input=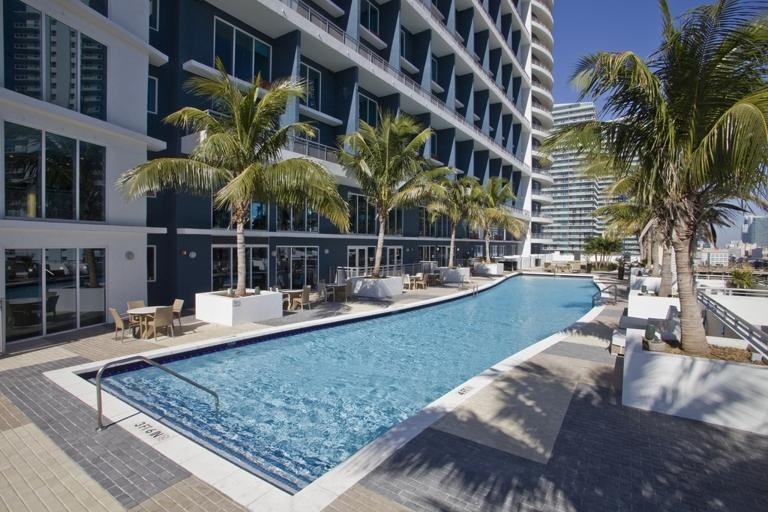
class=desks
[6,297,48,326]
[276,288,304,309]
[326,281,348,301]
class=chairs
[402,272,429,290]
[291,285,311,313]
[40,291,60,324]
[108,298,185,344]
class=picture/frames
[147,243,159,284]
